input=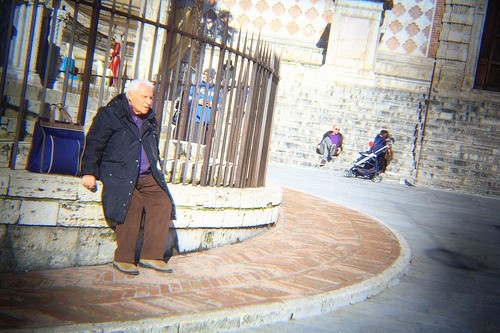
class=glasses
[334,128,340,131]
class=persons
[185,68,222,145]
[316,123,344,167]
[80,78,177,276]
[110,33,122,86]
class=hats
[204,68,216,78]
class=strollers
[343,129,395,183]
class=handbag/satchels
[27,103,85,177]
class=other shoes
[319,159,326,166]
[330,144,336,156]
[139,258,173,274]
[113,260,139,275]
